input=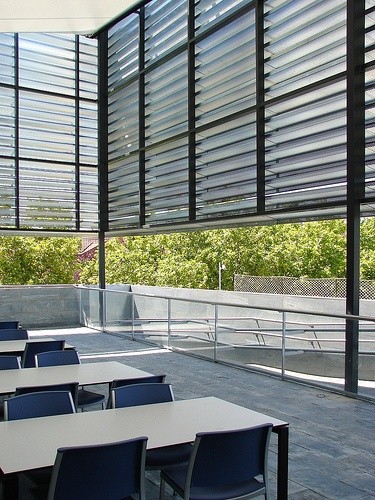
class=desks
[0,360,156,394]
[0,338,77,353]
[0,396,290,500]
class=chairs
[159,422,273,500]
[0,320,200,421]
[47,436,149,500]
